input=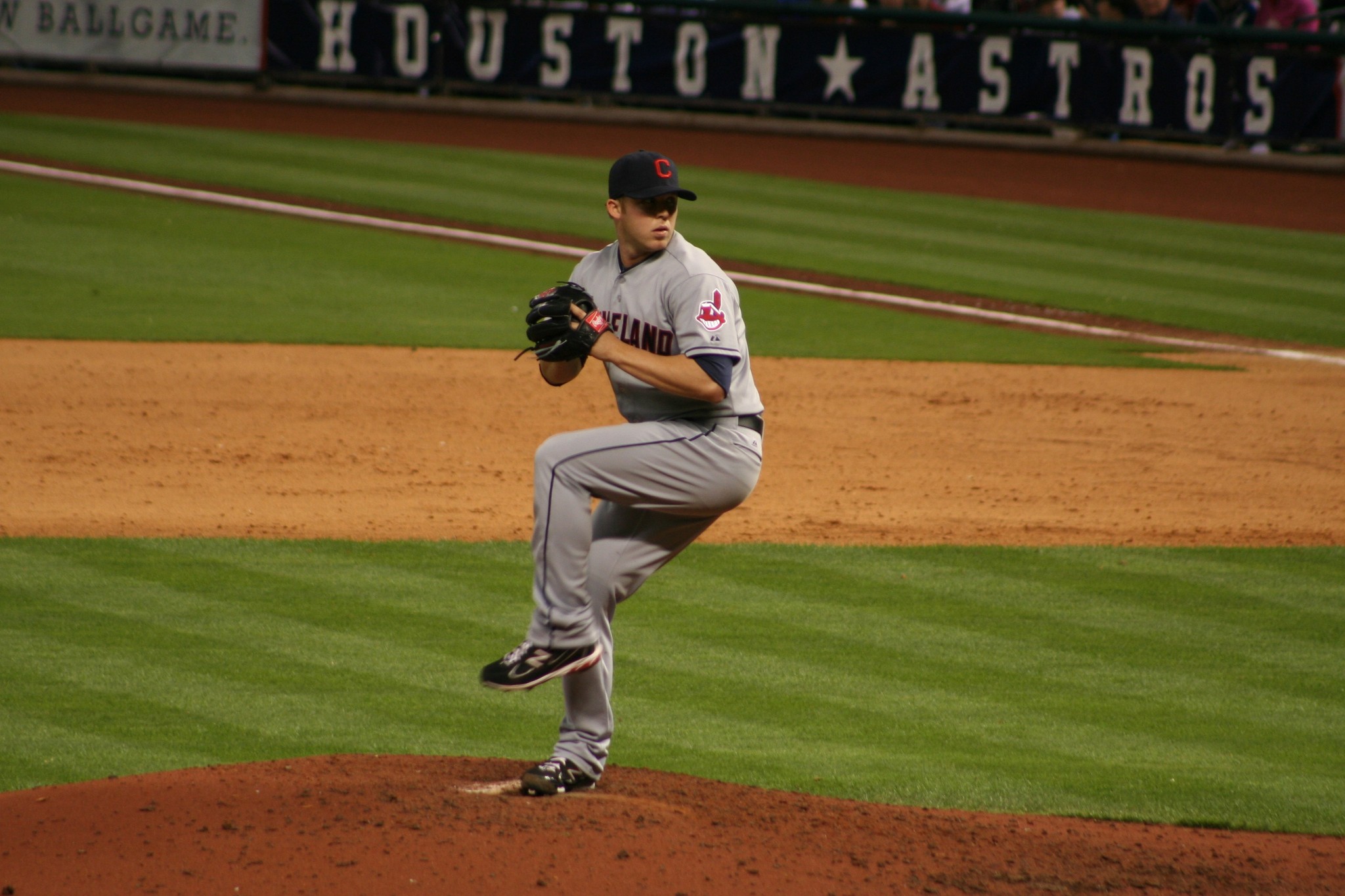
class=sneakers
[521,756,595,791]
[481,639,603,690]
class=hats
[609,149,697,201]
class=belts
[738,415,763,433]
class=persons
[479,146,763,795]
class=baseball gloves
[525,284,615,361]
